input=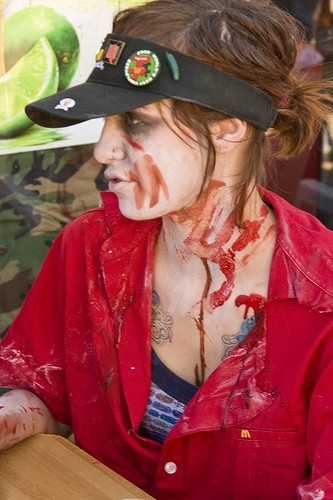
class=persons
[2,0,333,498]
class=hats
[24,33,278,133]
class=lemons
[0,5,80,139]
